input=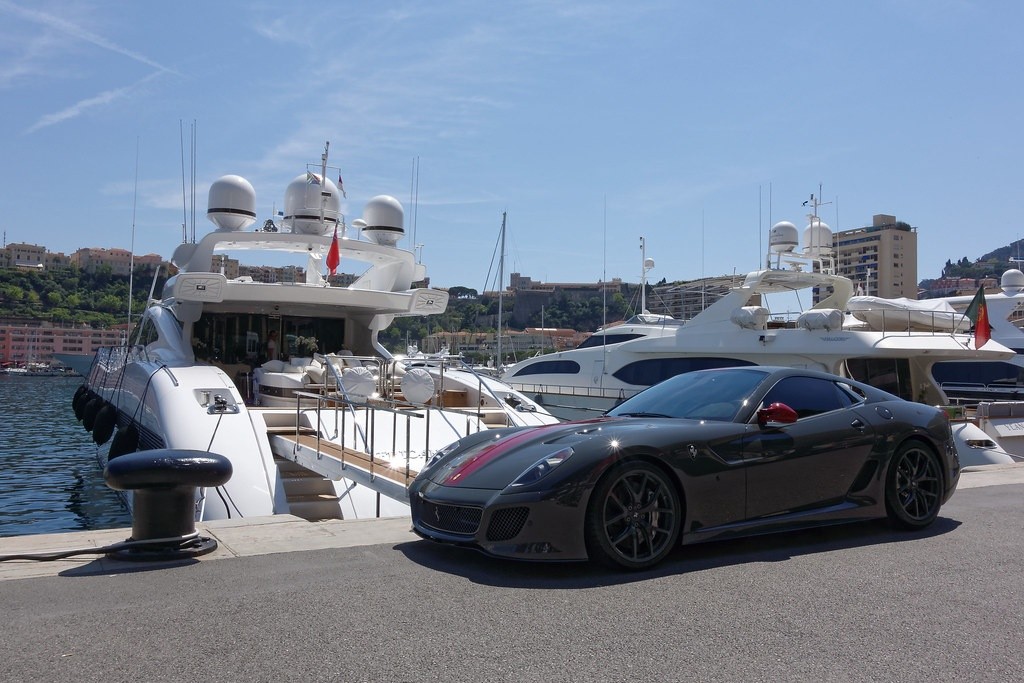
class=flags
[326,222,340,276]
[964,283,992,350]
[306,170,320,186]
[337,175,346,199]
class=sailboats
[409,212,543,376]
[4,316,50,376]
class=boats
[500,180,1023,463]
[73,135,563,521]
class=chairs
[253,368,304,390]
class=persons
[268,330,278,395]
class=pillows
[256,349,411,395]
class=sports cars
[406,364,962,569]
